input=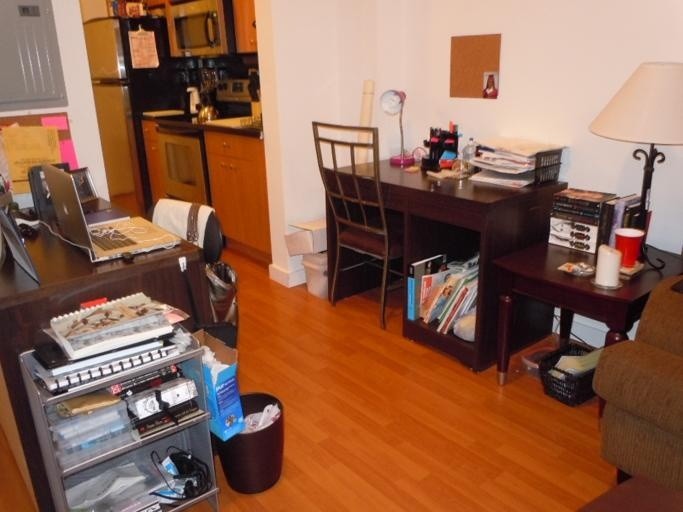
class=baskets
[539,341,596,406]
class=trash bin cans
[216,393,283,494]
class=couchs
[591,273,681,489]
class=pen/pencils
[429,121,459,138]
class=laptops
[42,162,181,263]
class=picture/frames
[70,167,100,204]
[28,162,70,220]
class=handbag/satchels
[203,261,238,327]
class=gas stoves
[155,107,246,129]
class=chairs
[144,199,225,263]
[311,120,403,330]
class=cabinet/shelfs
[141,121,170,202]
[19,322,220,511]
[204,129,272,256]
[233,1,257,55]
[473,142,562,188]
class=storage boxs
[183,328,246,442]
[302,251,328,297]
[285,218,326,256]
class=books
[29,289,193,392]
[407,252,479,336]
[549,187,645,261]
[472,133,567,189]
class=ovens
[155,125,209,206]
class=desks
[492,238,681,434]
[326,155,568,373]
[0,198,216,512]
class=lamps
[589,61,682,270]
[378,89,416,166]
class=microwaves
[165,0,231,58]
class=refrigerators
[81,14,188,225]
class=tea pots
[194,101,220,122]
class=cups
[613,226,646,268]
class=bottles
[460,136,476,173]
